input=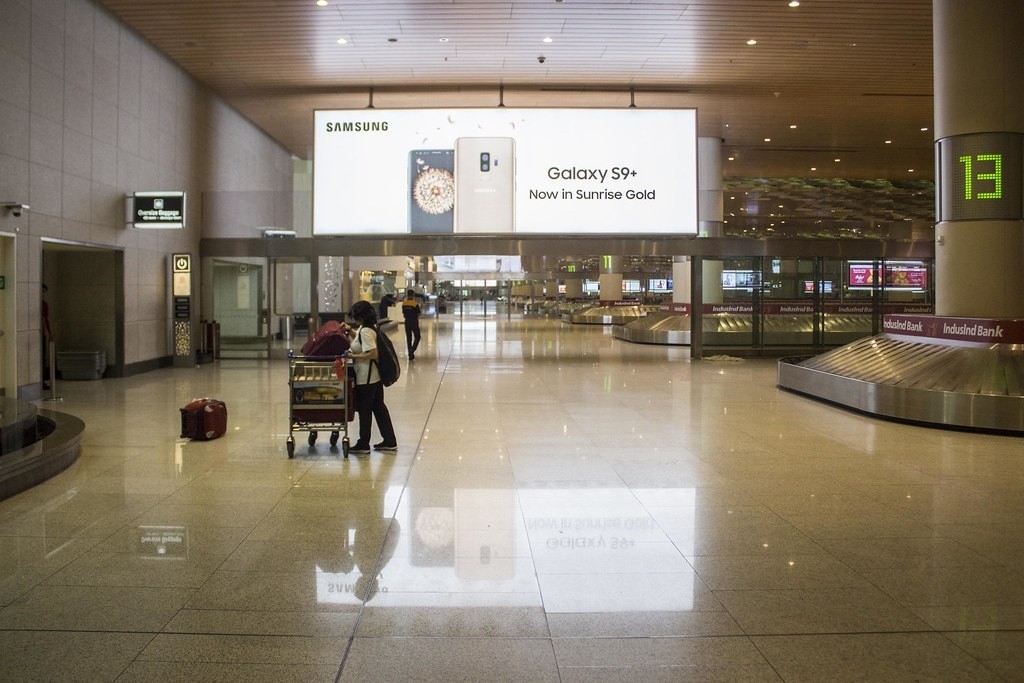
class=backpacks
[358,326,400,386]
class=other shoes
[409,346,414,353]
[409,355,415,360]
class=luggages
[179,397,227,440]
[300,320,351,362]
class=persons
[41,283,53,391]
[379,289,485,361]
[338,300,399,453]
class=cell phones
[408,149,455,233]
[453,137,516,235]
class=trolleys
[286,350,350,458]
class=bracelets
[345,324,353,332]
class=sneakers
[374,442,397,450]
[349,440,371,453]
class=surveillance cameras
[538,57,546,63]
[13,207,22,217]
